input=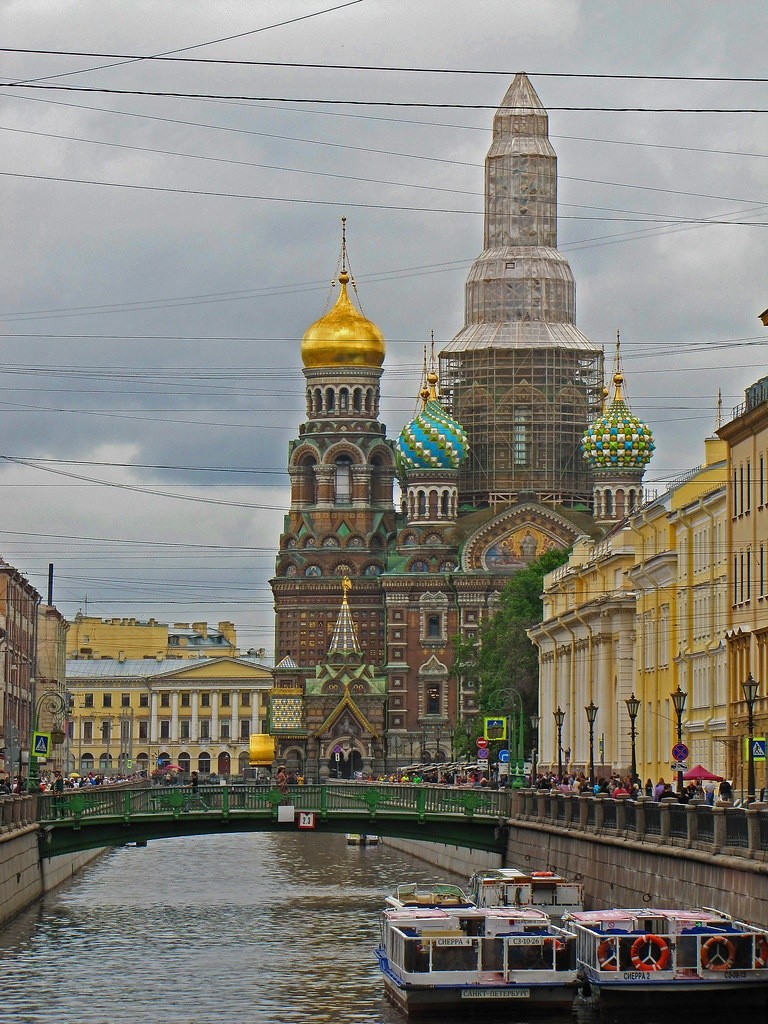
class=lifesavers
[597,936,631,971]
[530,871,554,877]
[630,934,670,971]
[741,934,768,969]
[700,935,736,971]
[543,937,561,949]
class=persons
[438,771,499,790]
[654,777,732,806]
[182,770,209,812]
[368,772,437,783]
[277,769,287,783]
[530,769,653,800]
[40,772,129,790]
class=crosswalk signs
[32,731,51,758]
[745,738,767,761]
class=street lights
[448,724,455,764]
[670,685,688,802]
[407,731,427,765]
[584,700,599,796]
[465,723,472,763]
[530,713,542,785]
[434,728,441,764]
[624,692,641,785]
[740,673,760,807]
[552,706,566,790]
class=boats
[345,834,379,845]
[561,906,768,1012]
[466,870,583,928]
[384,882,476,908]
[375,905,591,1014]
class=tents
[672,764,723,783]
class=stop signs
[476,737,488,748]
